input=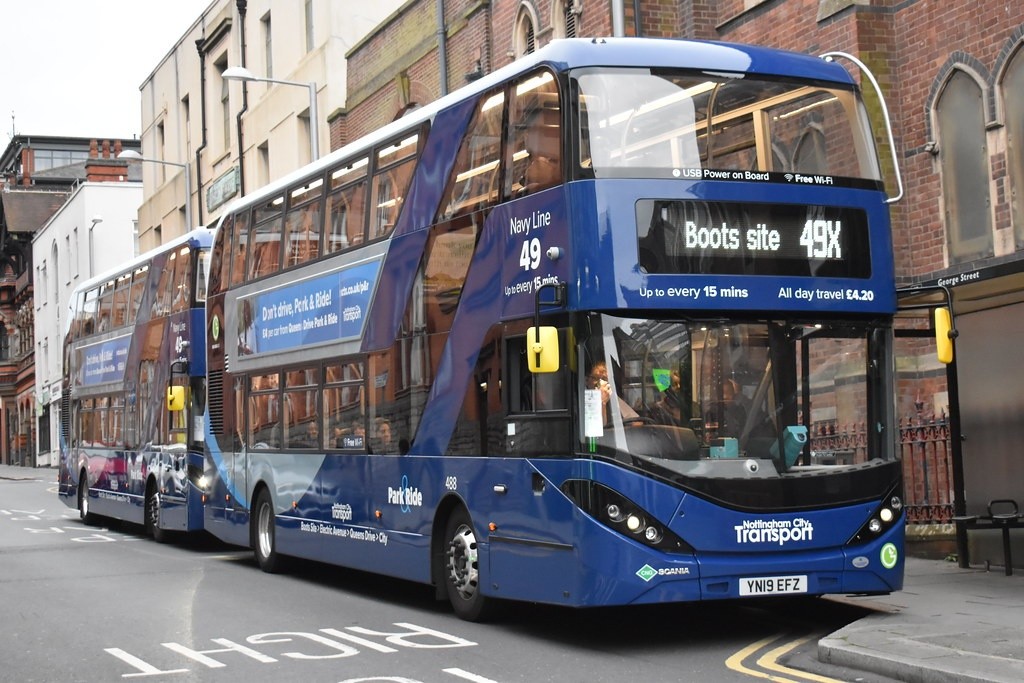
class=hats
[376,417,391,431]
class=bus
[201,33,961,624]
[59,228,218,544]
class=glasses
[588,374,608,382]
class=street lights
[221,66,319,163]
[116,150,192,233]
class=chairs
[234,224,392,284]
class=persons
[585,362,643,429]
[646,369,779,461]
[98,313,109,333]
[293,416,395,452]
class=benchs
[952,497,1024,577]
